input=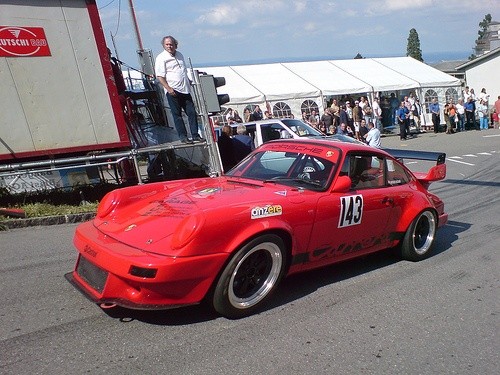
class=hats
[391,93,394,96]
[345,101,350,104]
[355,101,359,103]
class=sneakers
[193,135,206,143]
[180,136,193,144]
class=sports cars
[64,134,448,318]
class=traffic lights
[200,74,230,112]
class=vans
[201,118,327,173]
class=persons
[218,124,255,174]
[430,98,441,133]
[496,96,500,129]
[300,110,320,130]
[462,86,479,130]
[478,88,489,129]
[359,120,382,149]
[353,96,382,142]
[106,47,125,112]
[156,36,206,144]
[328,123,347,136]
[319,98,353,134]
[226,105,244,125]
[444,97,467,134]
[244,106,271,122]
[397,92,422,141]
[389,92,399,125]
